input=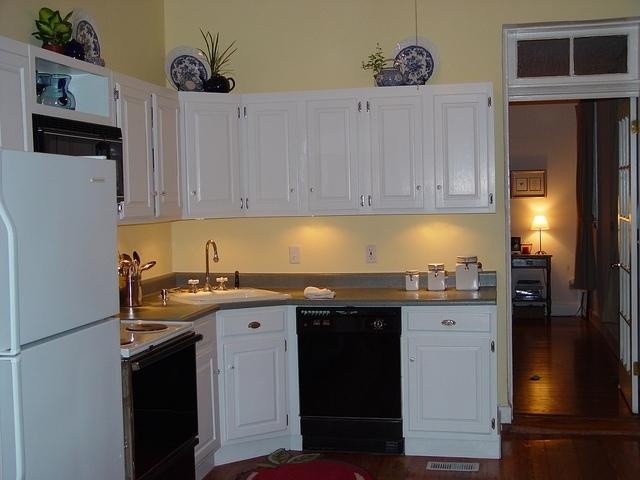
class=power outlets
[367,244,376,263]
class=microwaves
[31,118,125,204]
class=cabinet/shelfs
[301,86,429,215]
[428,82,496,215]
[193,305,303,480]
[178,91,301,218]
[29,43,117,127]
[110,71,182,225]
[0,35,33,151]
[400,305,502,460]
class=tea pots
[198,74,236,93]
[373,59,404,86]
[64,38,85,61]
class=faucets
[203,239,219,292]
[234,270,239,290]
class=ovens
[122,334,204,480]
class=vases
[36,73,75,110]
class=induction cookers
[120,316,193,358]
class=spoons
[118,248,157,275]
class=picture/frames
[509,169,547,199]
[511,237,521,251]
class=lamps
[531,215,550,255]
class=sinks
[158,286,290,304]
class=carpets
[234,458,373,480]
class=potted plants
[32,6,74,54]
[361,42,405,86]
[195,28,238,93]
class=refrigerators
[1,150,127,480]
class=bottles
[40,73,76,110]
[428,263,445,291]
[453,255,480,291]
[404,270,420,292]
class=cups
[120,274,143,308]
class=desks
[511,254,553,318]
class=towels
[304,287,336,298]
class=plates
[392,37,438,85]
[164,46,212,93]
[71,16,102,61]
